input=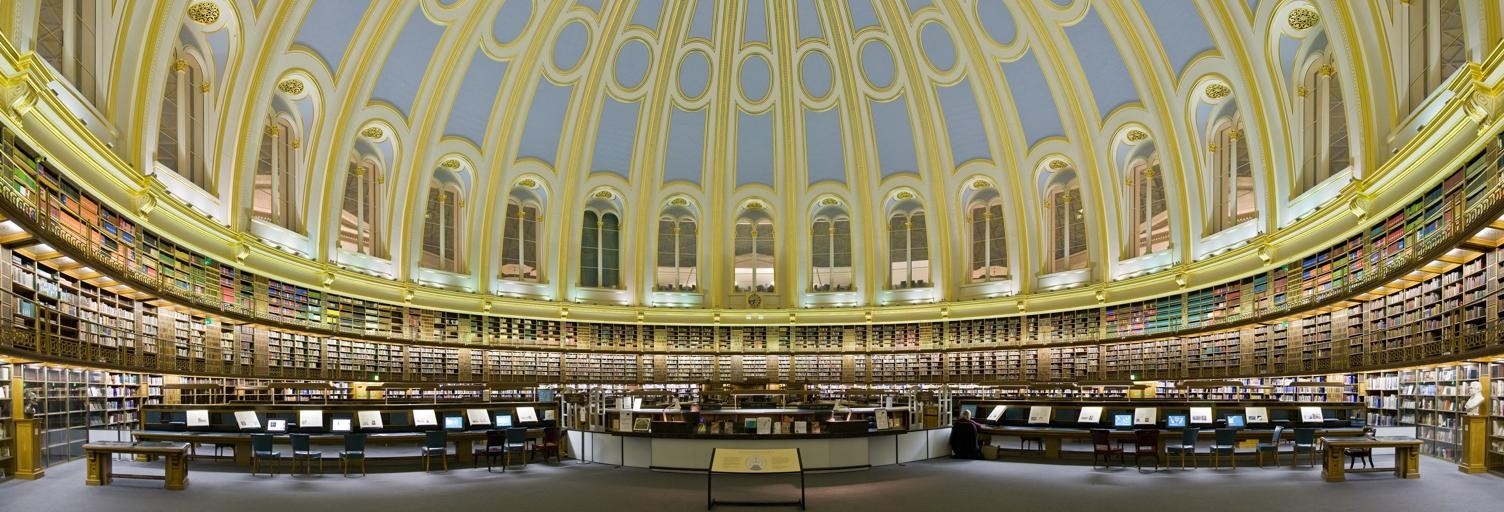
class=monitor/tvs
[494,413,513,429]
[960,404,977,419]
[1225,413,1246,431]
[266,418,288,435]
[443,415,466,432]
[1114,414,1133,430]
[1166,413,1187,432]
[331,417,353,435]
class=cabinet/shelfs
[377,304,1146,405]
[160,183,375,404]
[1,121,160,489]
[1145,207,1317,402]
[1317,128,1504,485]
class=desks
[83,406,375,489]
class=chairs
[249,425,563,477]
[945,419,1162,476]
[1146,398,1377,471]
